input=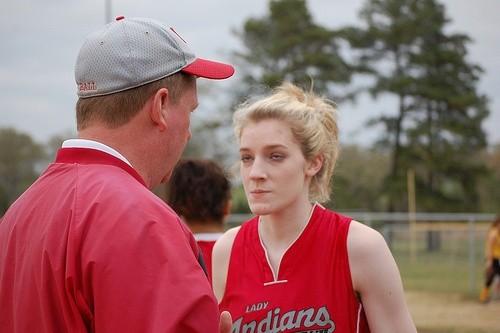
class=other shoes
[479,286,490,302]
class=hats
[74,16,235,99]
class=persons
[161,158,233,292]
[478,211,500,304]
[0,15,417,333]
